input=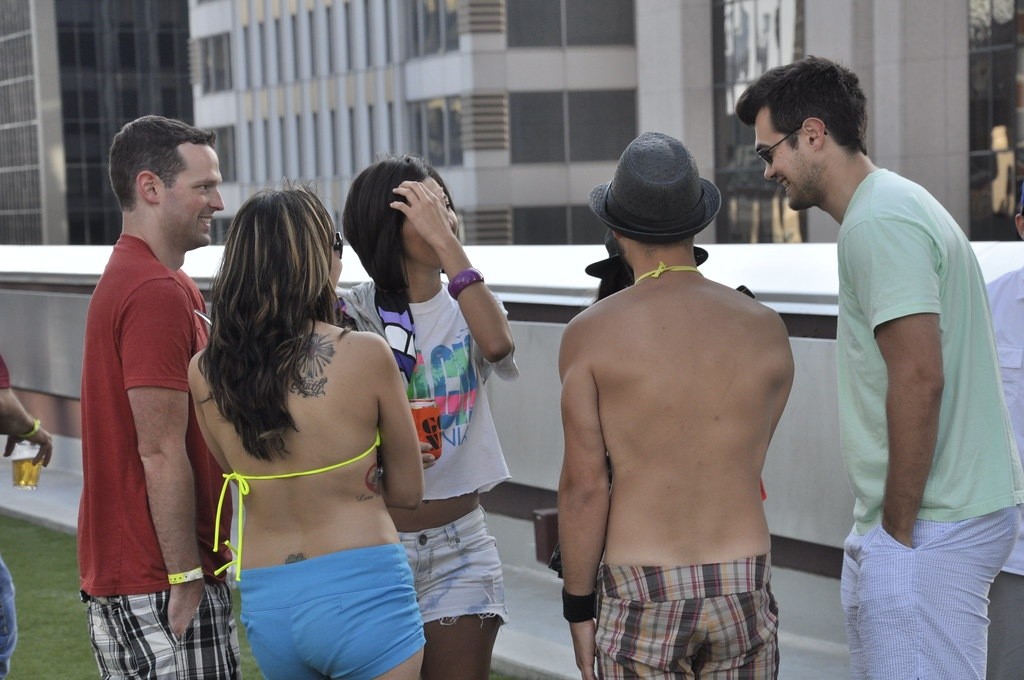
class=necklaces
[635,262,703,285]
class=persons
[189,178,425,680]
[0,346,53,680]
[78,115,242,680]
[335,155,519,680]
[736,54,1024,680]
[557,130,795,680]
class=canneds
[409,398,441,460]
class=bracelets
[448,268,485,300]
[18,418,41,438]
[561,587,596,623]
[168,567,203,585]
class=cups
[11,441,45,491]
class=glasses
[758,123,829,162]
[332,232,345,258]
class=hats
[583,226,710,281]
[587,131,722,244]
